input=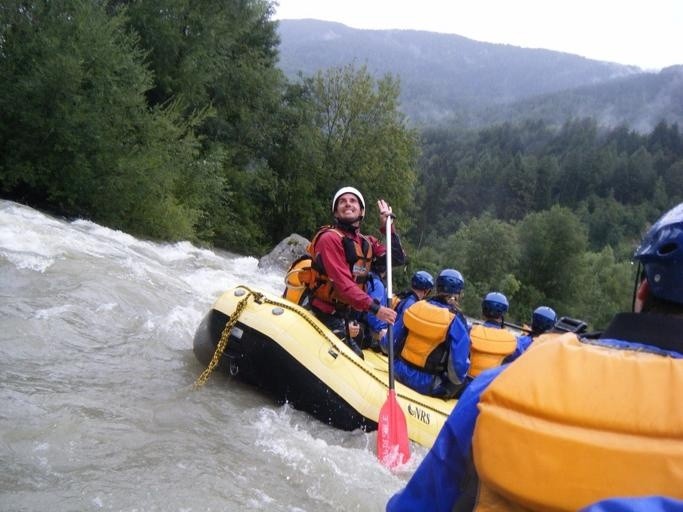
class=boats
[191,285,457,450]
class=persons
[393,269,471,399]
[453,292,519,395]
[517,306,558,355]
[365,258,385,339]
[285,243,360,337]
[378,271,434,358]
[308,186,406,359]
[385,201,683,512]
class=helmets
[483,292,509,318]
[412,270,434,290]
[332,187,366,215]
[533,306,557,331]
[634,202,683,303]
[436,269,464,294]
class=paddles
[377,212,410,469]
